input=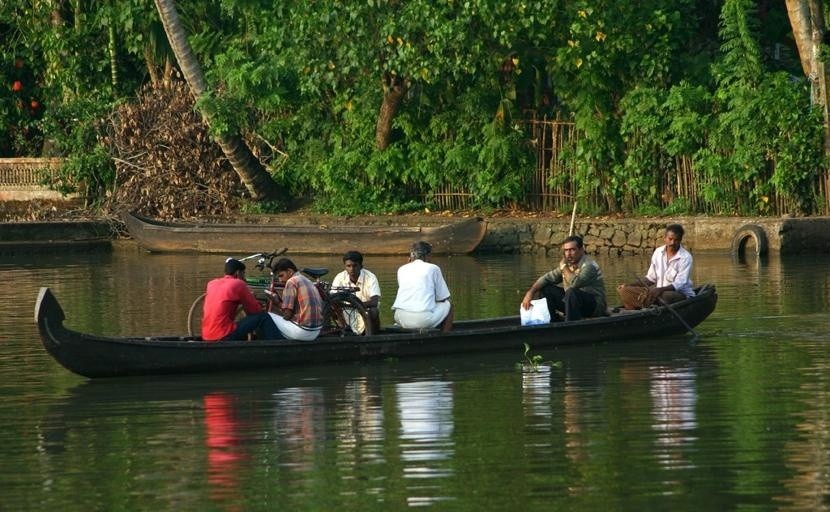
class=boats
[121,207,488,256]
[32,285,719,379]
[1,219,115,249]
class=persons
[202,259,280,341]
[267,258,324,341]
[523,238,607,322]
[330,251,379,336]
[619,224,695,307]
[391,241,454,333]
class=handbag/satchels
[519,297,552,327]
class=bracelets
[561,264,566,270]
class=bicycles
[187,246,369,336]
